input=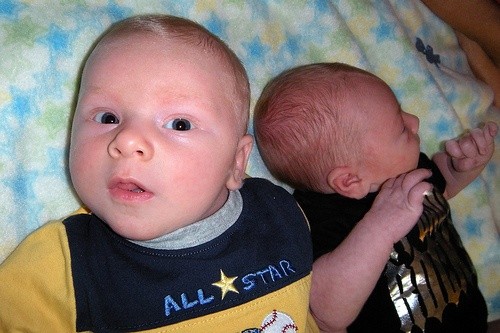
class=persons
[252,60,499,333]
[1,13,325,333]
